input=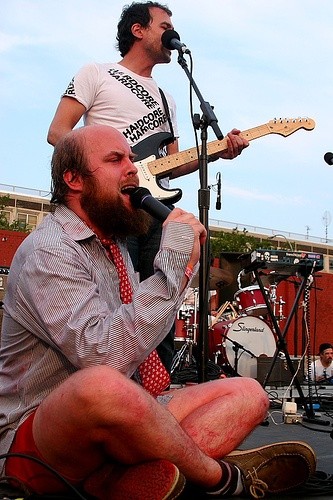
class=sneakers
[83,459,185,500]
[216,440,317,500]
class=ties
[80,216,173,399]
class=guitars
[128,115,316,206]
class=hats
[318,343,333,355]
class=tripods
[171,289,198,374]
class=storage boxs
[256,357,303,387]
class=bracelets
[185,267,193,279]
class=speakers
[256,357,304,385]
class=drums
[186,290,218,309]
[205,314,278,380]
[234,284,271,316]
[173,304,200,345]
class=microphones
[216,173,221,210]
[129,186,172,222]
[267,234,294,252]
[323,152,333,165]
[161,29,191,55]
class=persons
[49,0,250,388]
[307,343,333,381]
[0,123,317,500]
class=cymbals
[189,266,235,290]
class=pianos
[234,245,330,424]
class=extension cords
[283,413,304,424]
